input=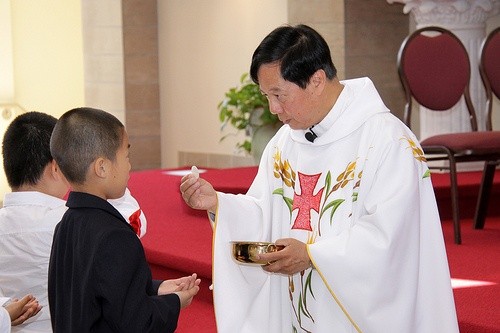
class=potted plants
[217,72,283,165]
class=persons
[0,291,43,332]
[48,107,202,331]
[180,24,423,333]
[2,112,150,332]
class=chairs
[396,26,500,243]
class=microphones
[304,128,318,143]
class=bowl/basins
[231,241,286,265]
